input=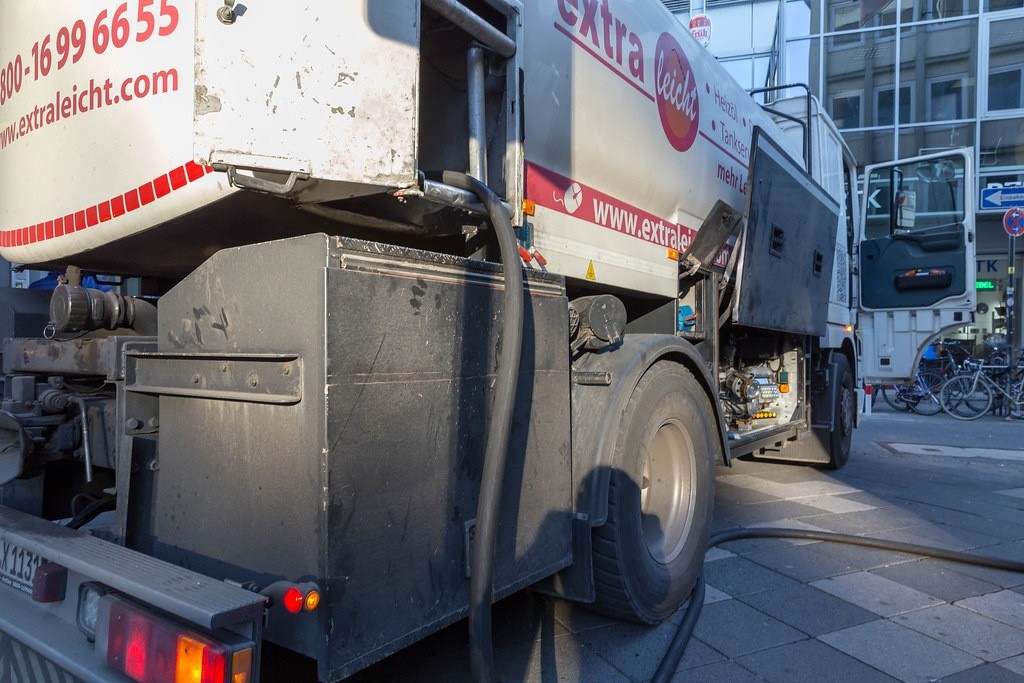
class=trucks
[0,0,984,683]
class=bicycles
[881,341,1024,421]
